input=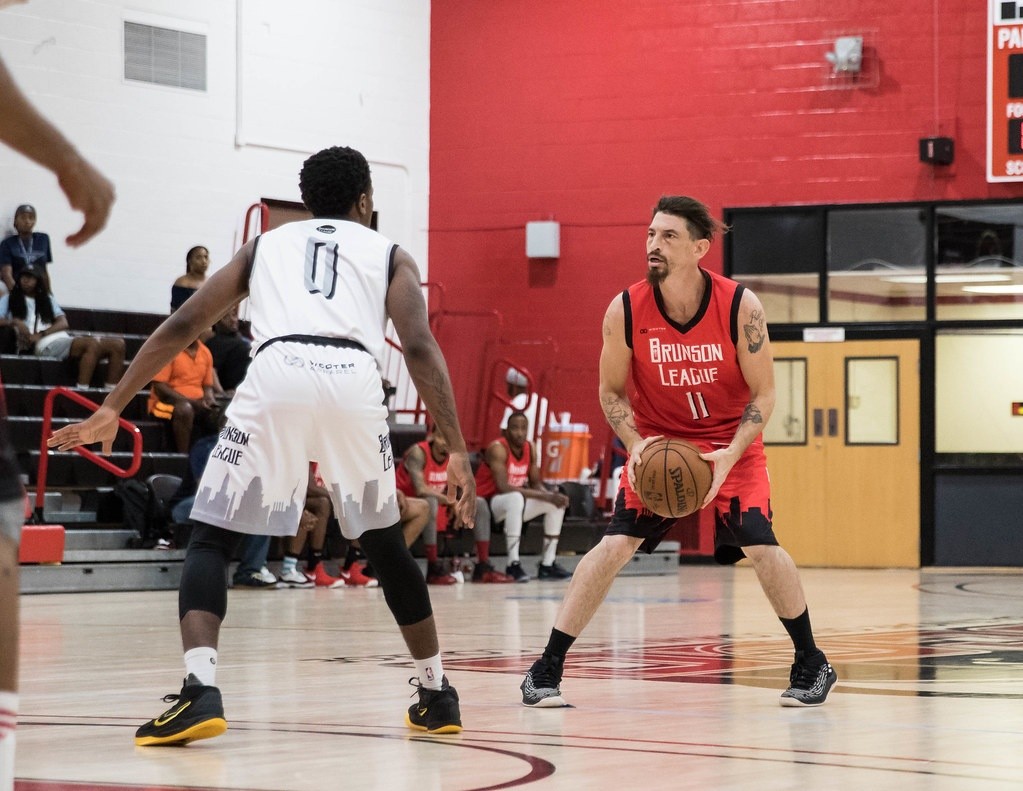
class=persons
[499,367,547,468]
[0,264,126,389]
[161,404,430,588]
[0,0,116,791]
[519,195,838,708]
[147,337,221,452]
[0,205,51,298]
[396,422,515,584]
[47,147,477,746]
[474,412,574,582]
[171,246,217,343]
[204,306,253,397]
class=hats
[17,264,43,278]
[13,205,37,228]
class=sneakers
[405,677,464,733]
[278,571,315,588]
[537,561,574,582]
[244,564,280,588]
[305,564,345,588]
[521,660,565,707]
[506,561,529,582]
[780,651,838,706]
[134,678,227,745]
[340,563,378,587]
[474,563,509,582]
[426,564,456,583]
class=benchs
[0,305,609,595]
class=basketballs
[632,438,715,520]
